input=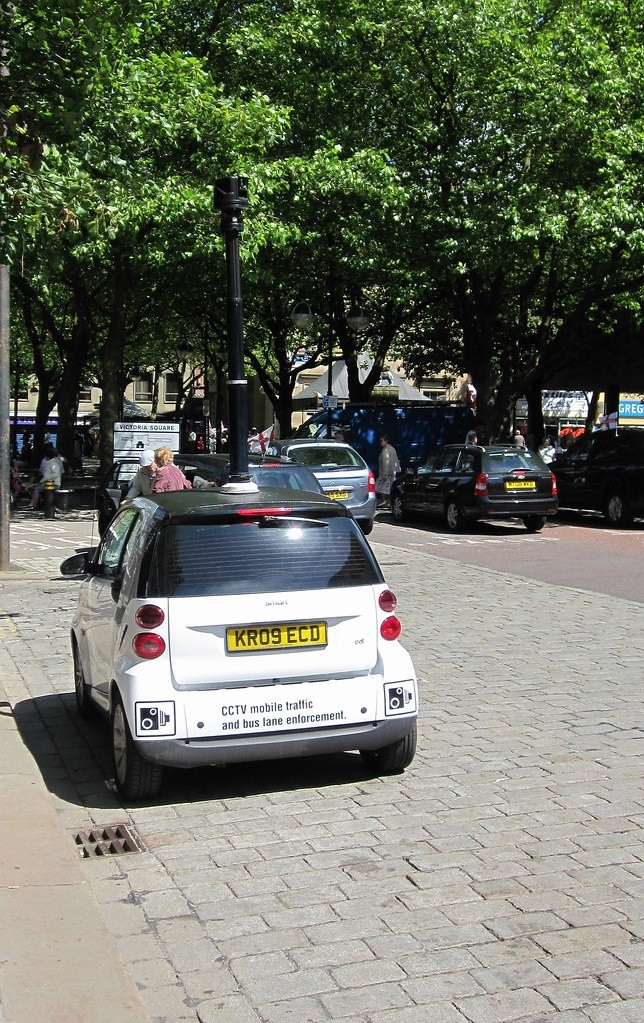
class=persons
[207,421,258,454]
[459,431,477,470]
[375,433,401,507]
[152,448,193,495]
[514,429,526,450]
[334,431,353,466]
[197,436,205,454]
[23,450,65,510]
[537,435,564,464]
[119,450,158,506]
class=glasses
[380,440,387,442]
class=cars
[60,481,421,803]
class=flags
[599,412,617,431]
[247,425,273,454]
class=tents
[292,346,436,411]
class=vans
[289,402,473,469]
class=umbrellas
[83,396,167,422]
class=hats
[138,450,155,467]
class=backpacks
[10,467,22,492]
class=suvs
[386,443,558,533]
[95,452,327,543]
[546,427,643,529]
[271,438,376,536]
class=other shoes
[378,500,391,507]
[23,504,35,510]
